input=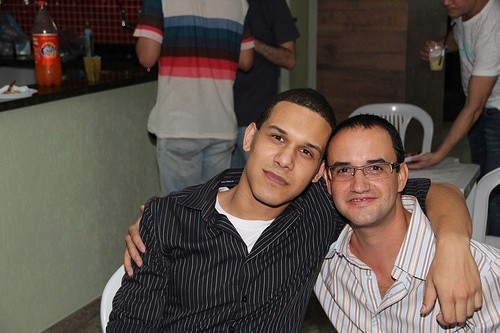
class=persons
[405,0,500,236]
[124,114,500,333]
[231,0,300,168]
[106,88,482,333]
[133,0,255,194]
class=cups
[428,41,445,70]
[84,57,101,81]
[15,37,28,56]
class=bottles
[83,22,94,57]
[32,0,60,88]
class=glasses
[327,162,399,181]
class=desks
[408,162,480,199]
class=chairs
[99,264,126,333]
[347,104,434,153]
